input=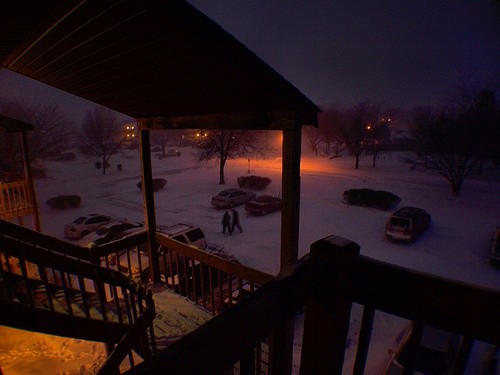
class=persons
[221,211,232,236]
[230,208,243,233]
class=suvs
[385,206,432,242]
[116,224,207,282]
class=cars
[44,150,76,162]
[64,214,164,265]
[211,188,257,210]
[490,227,500,265]
[244,195,282,216]
[385,321,463,375]
